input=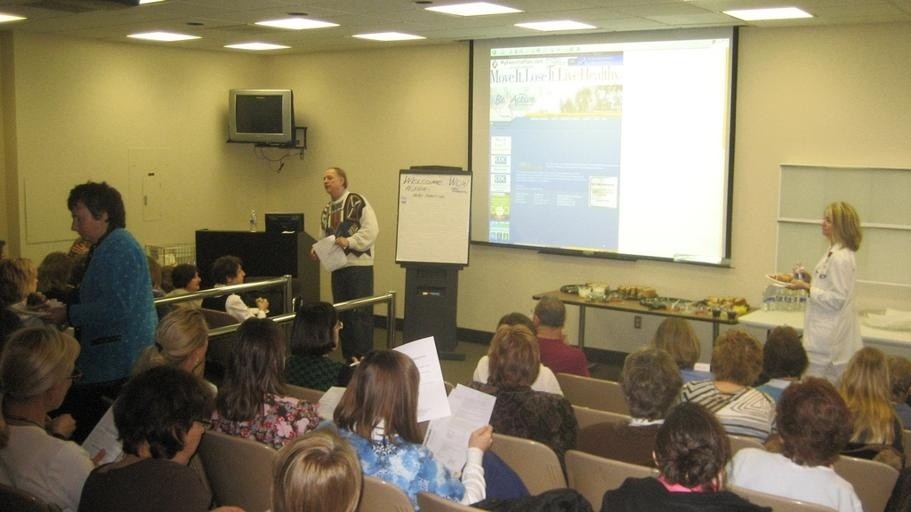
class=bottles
[795,262,804,283]
[249,209,257,232]
[764,290,807,312]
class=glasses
[62,367,83,385]
[335,322,344,331]
[194,418,213,430]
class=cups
[712,303,736,321]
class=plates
[10,302,64,316]
[765,274,794,288]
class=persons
[310,167,379,365]
[785,201,865,387]
[39,182,160,452]
[1,235,910,512]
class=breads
[619,286,656,298]
[775,273,793,283]
[705,297,747,311]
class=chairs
[417,491,477,511]
[833,451,898,512]
[553,370,632,415]
[199,430,280,512]
[282,382,326,403]
[724,435,766,462]
[489,433,566,496]
[357,474,413,512]
[725,483,834,511]
[568,403,634,430]
[564,448,659,511]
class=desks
[533,290,757,369]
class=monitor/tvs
[228,88,296,145]
[264,213,304,233]
